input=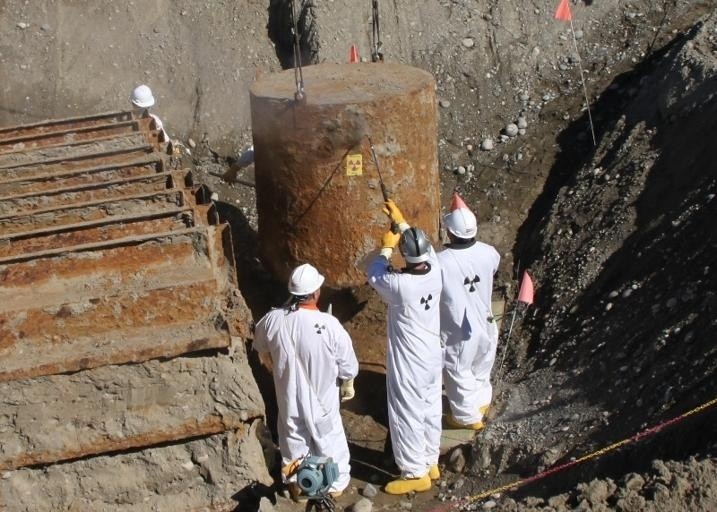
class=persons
[430,207,501,431]
[365,198,442,495]
[132,84,170,144]
[251,264,359,503]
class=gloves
[381,198,407,225]
[380,230,400,250]
[258,351,274,373]
[337,379,356,403]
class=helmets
[286,263,324,295]
[442,209,477,240]
[398,228,431,265]
[129,84,156,110]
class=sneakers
[479,404,488,419]
[329,492,340,497]
[427,464,440,480]
[384,476,431,494]
[446,414,483,430]
[291,484,307,503]
